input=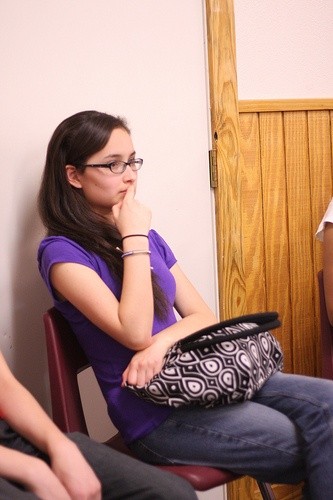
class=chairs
[42,305,276,500]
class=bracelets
[121,234,149,241]
[121,251,151,258]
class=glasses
[78,157,143,174]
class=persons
[37,111,333,500]
[314,200,333,326]
[0,354,200,500]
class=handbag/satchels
[122,312,285,408]
